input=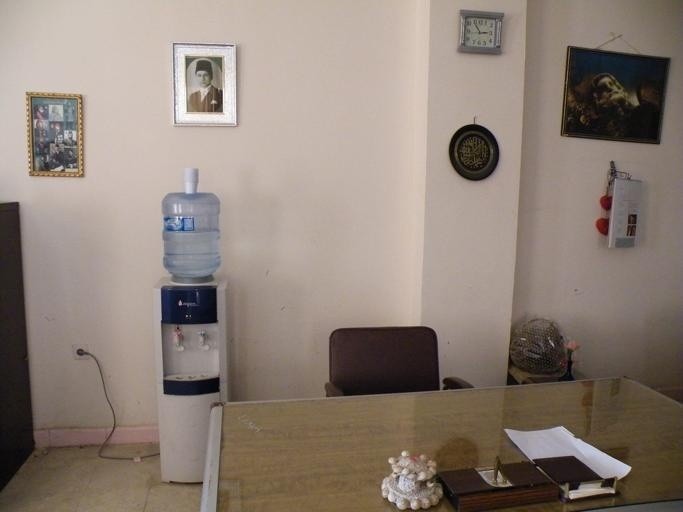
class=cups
[184,168,198,194]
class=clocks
[457,10,504,55]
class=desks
[199,376,682,511]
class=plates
[448,124,500,181]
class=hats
[195,60,212,71]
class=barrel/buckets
[162,191,222,280]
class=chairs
[325,327,474,396]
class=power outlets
[73,342,91,360]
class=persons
[33,104,78,172]
[187,60,222,112]
[592,74,661,140]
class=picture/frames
[561,46,670,145]
[172,42,237,128]
[26,91,84,178]
